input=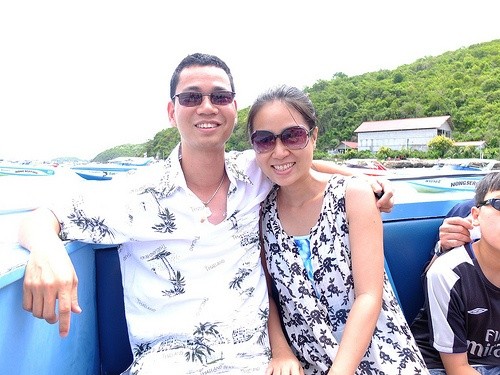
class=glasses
[171,90,237,107]
[476,198,500,211]
[250,125,316,154]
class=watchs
[434,240,442,256]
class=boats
[344,158,500,196]
[0,156,156,181]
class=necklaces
[179,158,227,217]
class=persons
[408,173,500,375]
[17,52,393,375]
[246,84,432,375]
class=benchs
[381,198,500,375]
